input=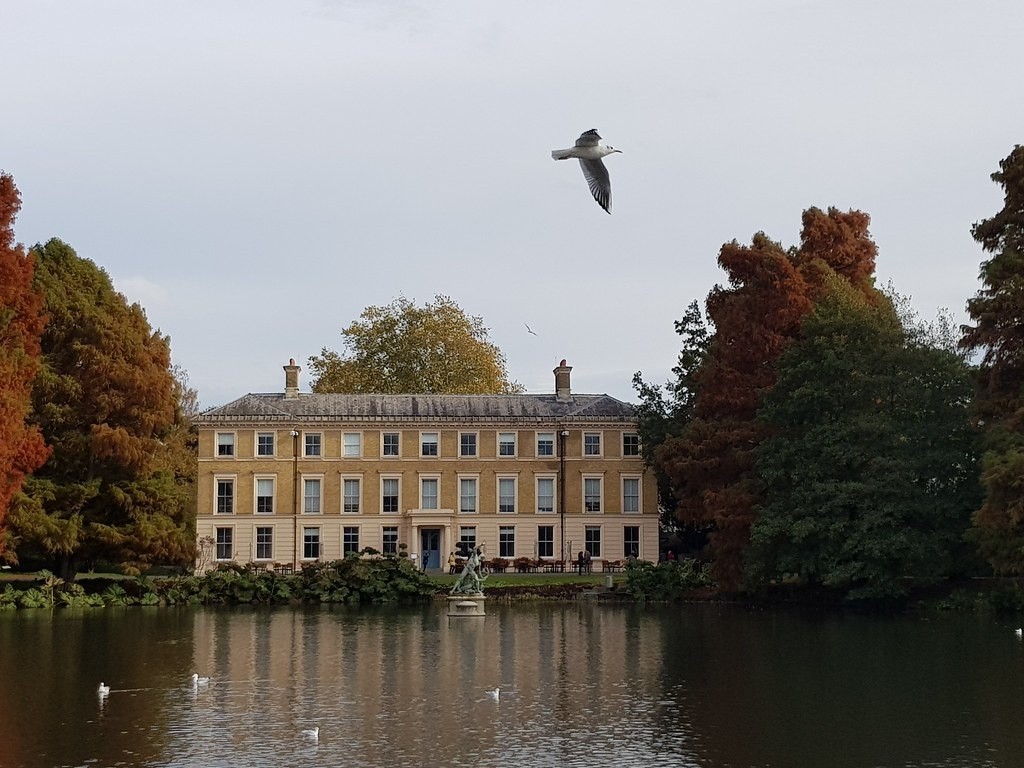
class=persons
[577,549,591,576]
[628,550,674,571]
[449,552,456,575]
[422,551,429,571]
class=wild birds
[1015,627,1022,635]
[551,128,622,215]
[301,727,319,737]
[525,324,537,335]
[192,673,211,682]
[485,688,500,698]
[97,682,110,694]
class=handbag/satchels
[448,558,450,563]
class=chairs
[245,563,267,574]
[450,557,593,574]
[274,562,294,575]
[602,560,622,573]
